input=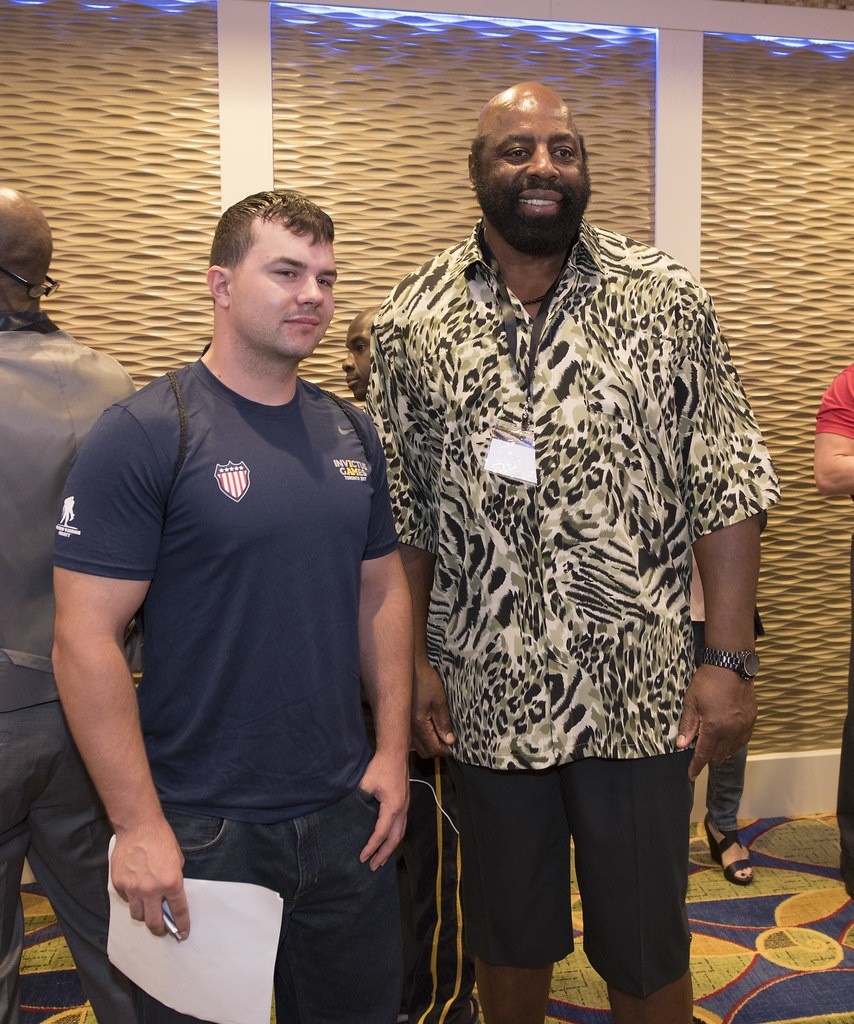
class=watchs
[700,645,759,681]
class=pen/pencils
[162,909,183,941]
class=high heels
[704,813,753,886]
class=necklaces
[521,295,546,306]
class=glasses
[0,266,60,299]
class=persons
[0,187,414,1023]
[815,361,854,900]
[342,82,780,1023]
